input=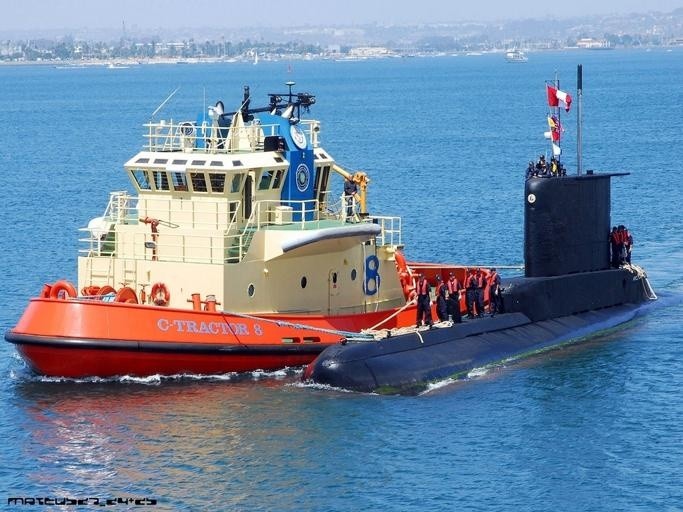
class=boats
[301,64,653,396]
[504,48,527,63]
[4,62,493,379]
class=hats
[418,268,496,280]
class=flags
[546,85,571,113]
[547,114,566,142]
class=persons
[343,174,358,222]
[524,155,566,181]
[610,224,634,269]
[415,267,501,329]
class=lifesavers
[395,251,406,272]
[400,273,417,304]
[50,280,77,300]
[150,283,170,305]
